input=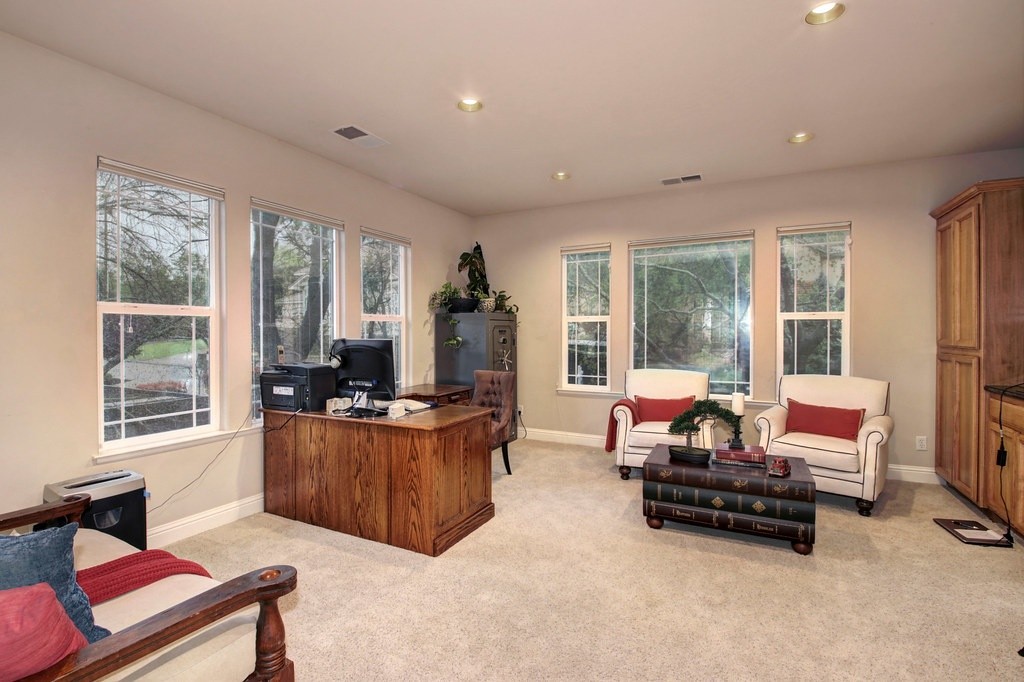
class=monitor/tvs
[329,340,398,417]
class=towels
[605,398,642,453]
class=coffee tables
[641,442,818,557]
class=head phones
[329,338,347,369]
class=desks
[257,404,496,558]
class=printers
[260,362,336,412]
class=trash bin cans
[43,470,147,551]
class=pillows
[633,394,696,423]
[785,396,867,442]
[0,521,113,642]
[0,580,88,682]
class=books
[711,442,767,468]
[375,399,432,411]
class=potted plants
[667,400,744,462]
[426,282,480,348]
[473,287,520,316]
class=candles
[732,392,746,415]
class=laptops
[933,518,1013,548]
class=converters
[996,450,1006,466]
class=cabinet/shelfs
[396,383,475,405]
[929,175,1024,544]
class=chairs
[467,369,519,476]
[0,494,298,682]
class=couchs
[614,369,718,482]
[757,374,896,512]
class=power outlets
[518,404,524,416]
[916,436,927,451]
